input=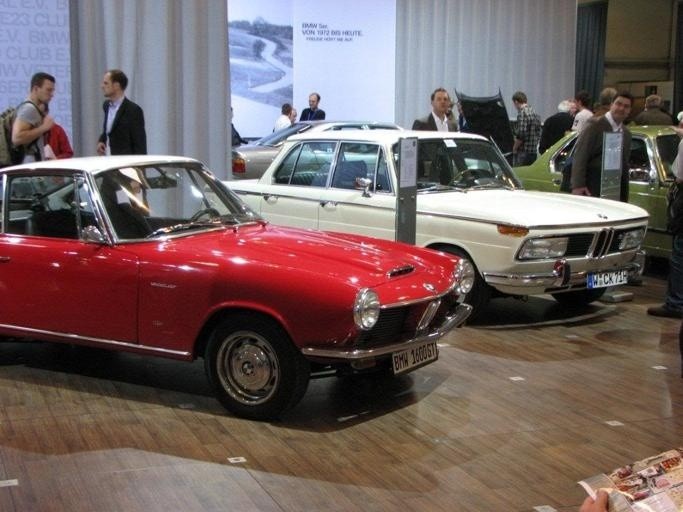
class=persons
[299,92,325,121]
[97,68,148,206]
[446,96,458,131]
[635,94,675,125]
[570,90,634,203]
[274,102,292,132]
[537,100,578,154]
[645,110,683,321]
[511,91,542,168]
[230,105,249,147]
[458,103,472,132]
[572,92,595,131]
[290,109,297,124]
[411,88,475,185]
[592,84,618,115]
[39,102,74,160]
[10,71,55,198]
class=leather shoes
[647,303,683,318]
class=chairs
[310,159,370,189]
[23,206,76,238]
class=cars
[232,121,405,181]
[199,129,650,312]
[0,156,475,417]
[509,125,683,275]
[454,88,546,162]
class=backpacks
[0,100,45,168]
